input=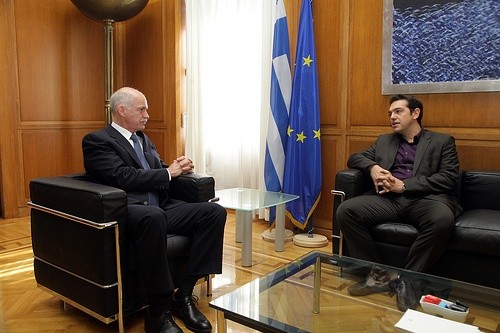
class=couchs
[27,174,215,333]
[332,169,500,289]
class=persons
[82,86,228,333]
[336,94,464,313]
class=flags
[282,0,323,236]
[263,0,294,235]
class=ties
[131,134,150,170]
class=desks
[208,188,300,267]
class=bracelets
[402,183,407,191]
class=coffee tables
[209,249,500,333]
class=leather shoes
[172,294,212,333]
[144,311,183,333]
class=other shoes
[394,280,418,311]
[348,270,392,296]
